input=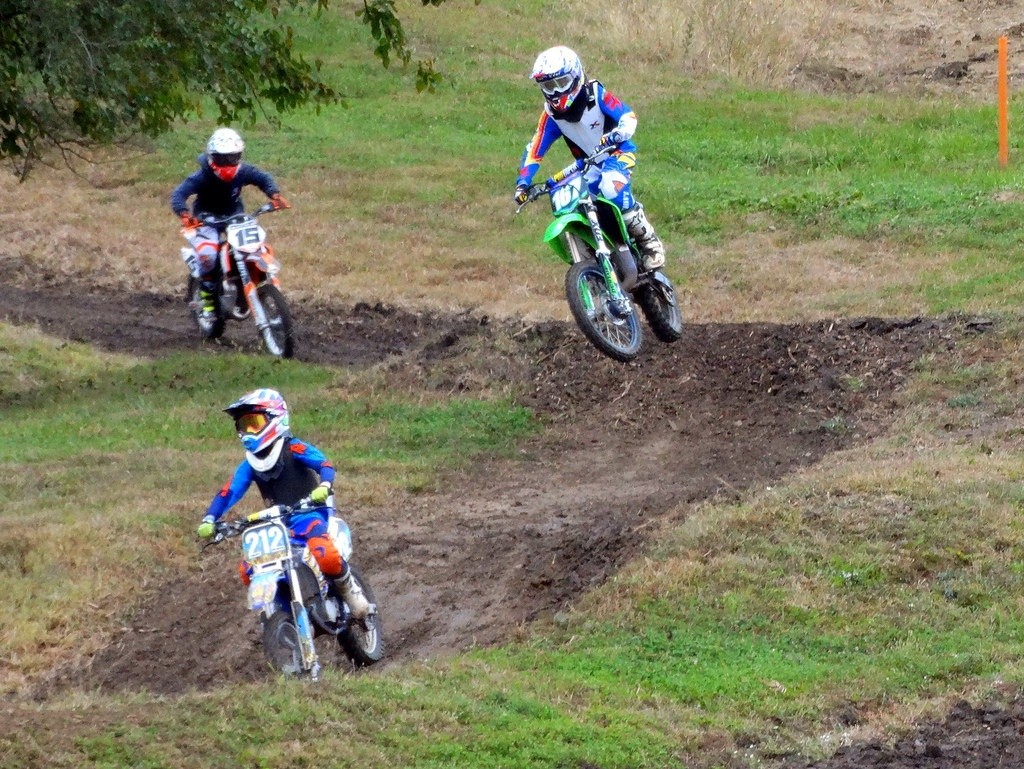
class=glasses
[237,412,270,435]
[539,75,575,96]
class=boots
[330,567,368,620]
[622,209,664,270]
[198,284,220,331]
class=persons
[514,46,665,268]
[170,128,289,322]
[198,388,369,619]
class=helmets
[207,127,244,179]
[219,389,288,454]
[532,46,585,112]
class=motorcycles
[517,132,684,361]
[197,487,386,679]
[179,199,297,360]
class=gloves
[310,486,330,502]
[516,185,535,205]
[272,193,290,210]
[179,212,200,228]
[197,521,214,537]
[599,128,627,148]
